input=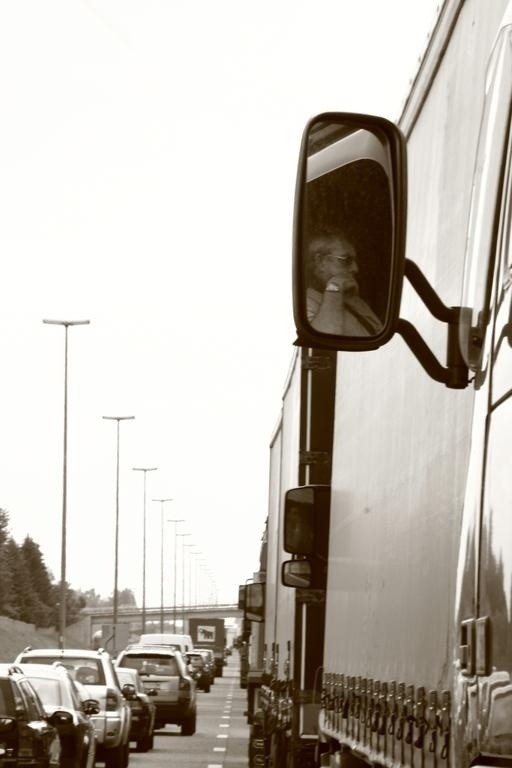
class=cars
[0,617,233,768]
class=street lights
[102,415,207,649]
[43,319,90,649]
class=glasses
[327,254,357,263]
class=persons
[306,223,384,337]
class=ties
[342,303,376,336]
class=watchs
[326,283,344,295]
